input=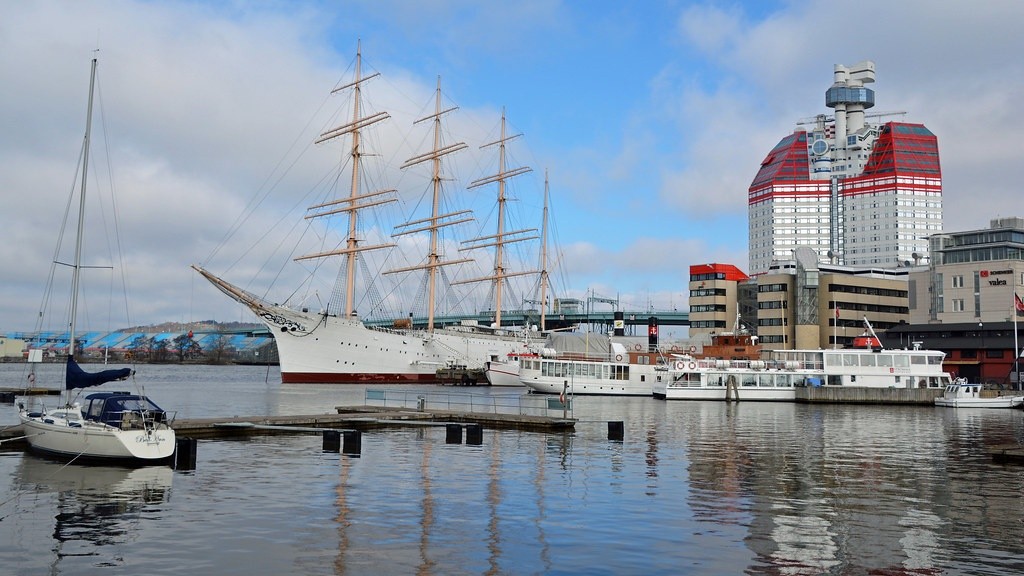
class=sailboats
[189,33,627,384]
[13,44,179,460]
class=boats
[652,350,952,404]
[484,360,526,388]
[934,377,1024,408]
[507,330,666,397]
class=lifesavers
[635,344,642,351]
[616,354,622,362]
[677,362,685,369]
[689,345,696,352]
[671,345,678,352]
[689,362,696,369]
[560,392,564,403]
